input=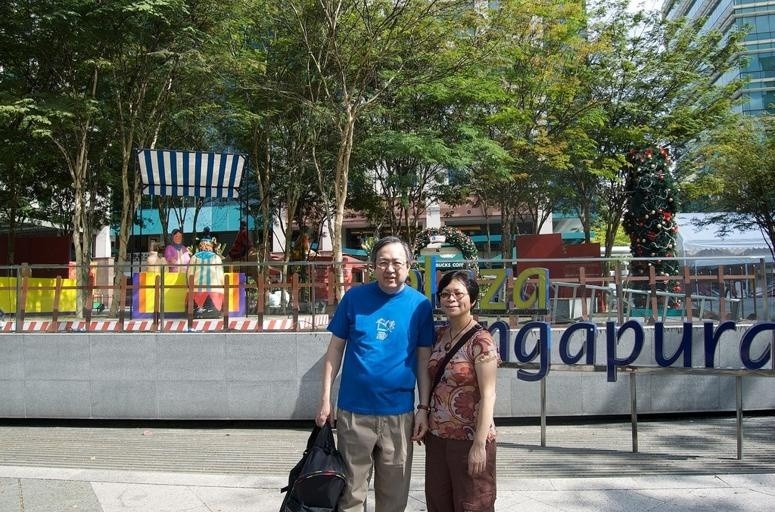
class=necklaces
[442,319,474,351]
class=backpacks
[280,421,346,512]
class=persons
[416,269,501,512]
[292,234,316,254]
[163,228,191,272]
[203,226,211,239]
[314,236,436,511]
[182,241,225,319]
[227,219,254,260]
[264,279,289,308]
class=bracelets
[417,404,432,413]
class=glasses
[439,291,469,299]
[375,261,410,268]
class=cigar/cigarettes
[417,440,421,445]
